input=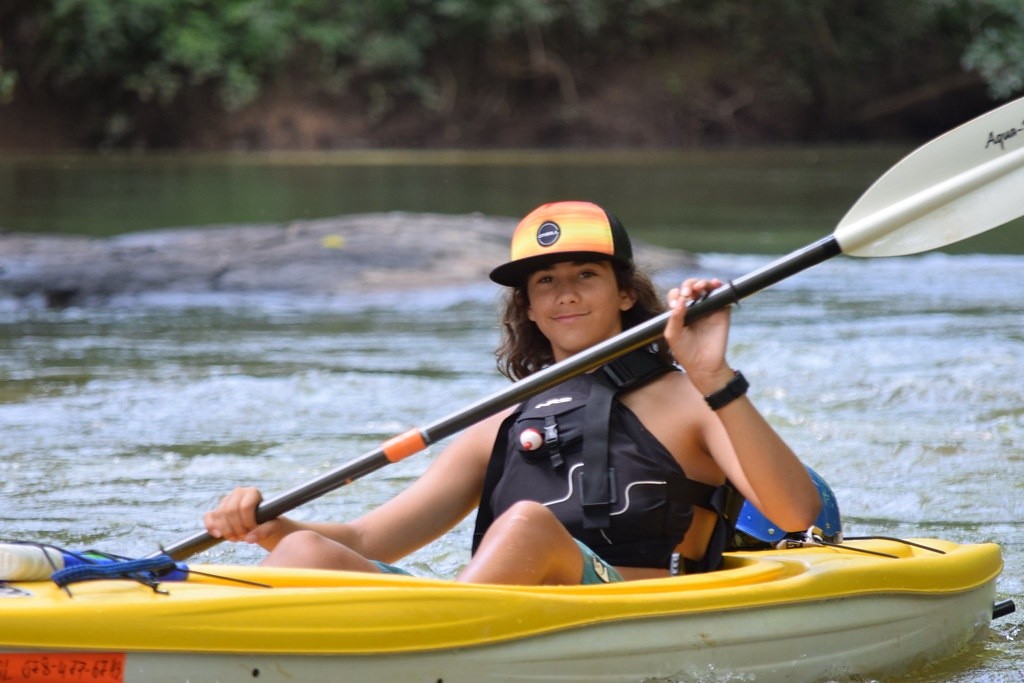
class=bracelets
[705,371,749,411]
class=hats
[490,201,633,287]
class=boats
[0,536,1017,683]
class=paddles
[152,88,1024,585]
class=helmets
[736,465,843,548]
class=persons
[205,200,821,584]
